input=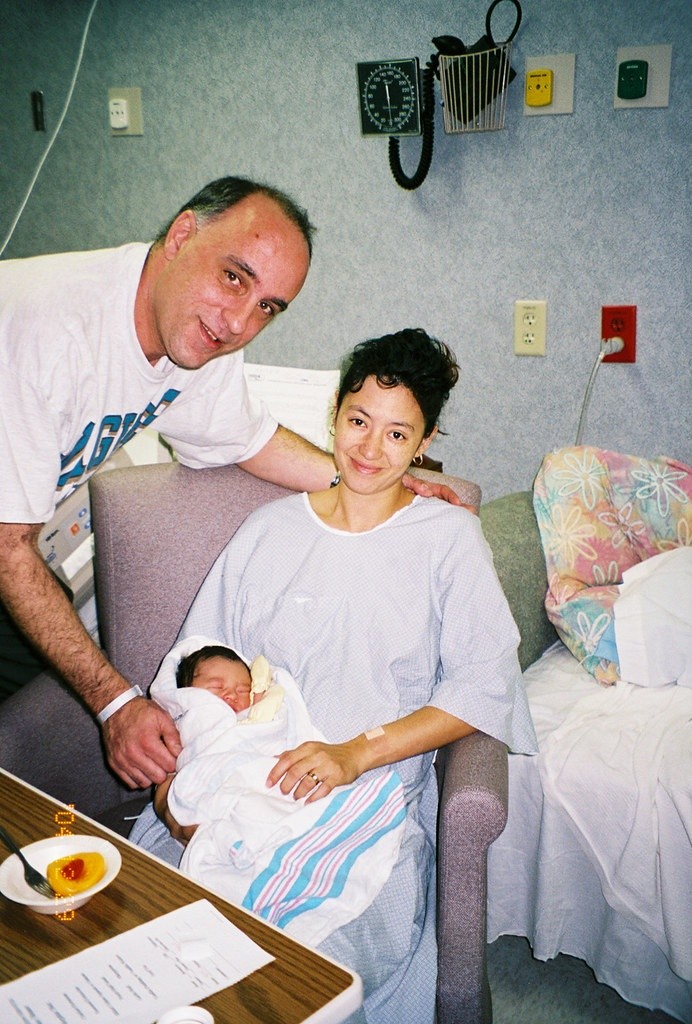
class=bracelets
[331,469,341,488]
[95,684,143,726]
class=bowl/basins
[0,834,123,914]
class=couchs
[477,443,692,1024]
[0,462,509,1024]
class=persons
[0,177,539,1024]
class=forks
[0,826,57,898]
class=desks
[0,771,364,1024]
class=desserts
[46,852,104,895]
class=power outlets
[601,305,637,363]
[108,87,144,136]
[514,300,546,357]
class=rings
[307,772,321,785]
